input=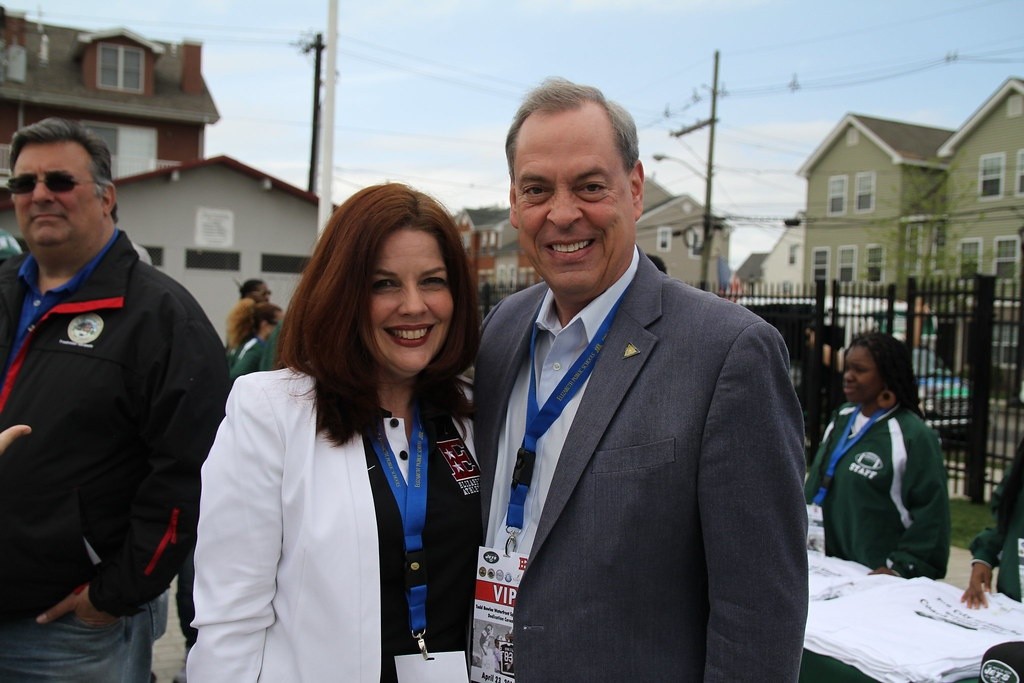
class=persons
[225,277,1024,610]
[0,424,32,455]
[186,183,482,683]
[467,78,809,683]
[0,119,229,683]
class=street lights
[653,51,721,292]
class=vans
[731,298,968,462]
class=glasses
[7,170,94,194]
